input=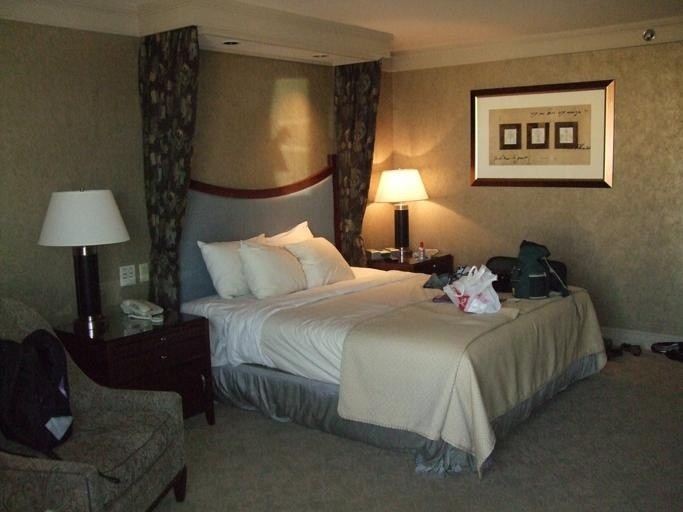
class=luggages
[486,256,566,292]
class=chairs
[0,297,187,512]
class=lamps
[373,167,428,257]
[36,188,131,329]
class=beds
[175,154,590,469]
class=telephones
[119,298,165,317]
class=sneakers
[606,347,622,357]
[622,343,641,355]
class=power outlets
[119,265,136,288]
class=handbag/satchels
[510,240,552,297]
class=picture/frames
[470,79,615,187]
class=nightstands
[367,252,452,279]
[51,304,215,426]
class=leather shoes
[651,342,683,362]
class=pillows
[195,219,354,300]
